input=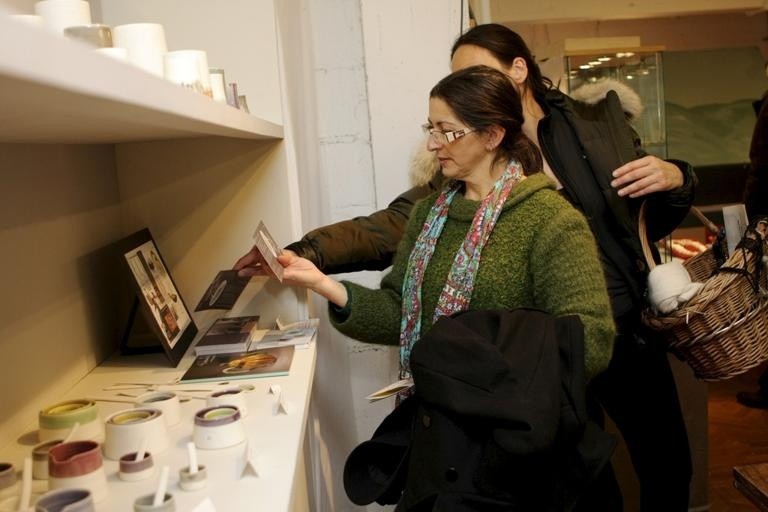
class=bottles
[226,82,249,112]
[0,384,248,511]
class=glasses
[420,122,483,147]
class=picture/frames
[107,227,199,368]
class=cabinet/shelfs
[1,0,321,511]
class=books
[177,343,296,384]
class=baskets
[641,193,768,384]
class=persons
[231,21,697,512]
[277,65,625,511]
[734,85,768,413]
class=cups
[34,0,92,34]
[166,49,212,93]
[112,22,169,78]
[209,71,226,104]
[63,23,114,52]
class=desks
[733,461,768,512]
